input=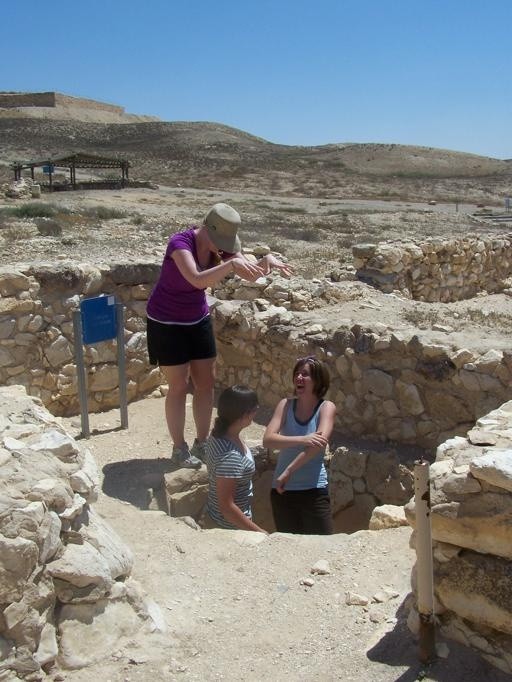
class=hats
[205,203,241,254]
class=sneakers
[171,443,202,468]
[190,438,207,464]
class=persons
[263,356,336,533]
[144,203,294,469]
[203,387,264,532]
